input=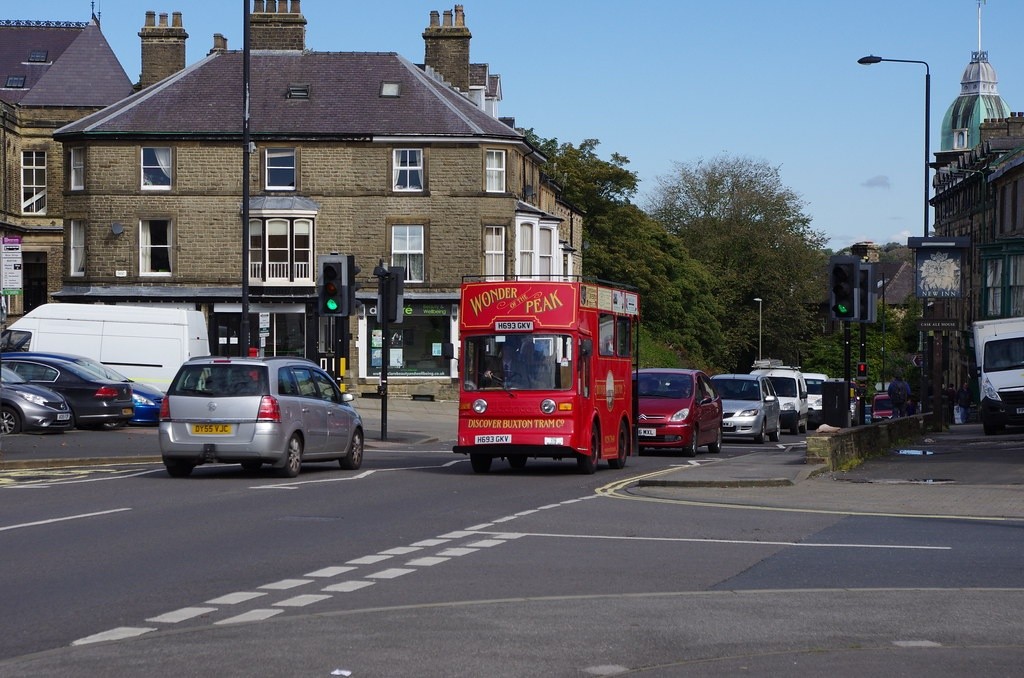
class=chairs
[203,375,225,391]
[237,375,254,392]
[649,379,679,392]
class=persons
[677,379,691,397]
[948,383,974,425]
[484,342,547,388]
[637,379,653,394]
[886,370,917,419]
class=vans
[801,371,828,426]
[0,302,210,394]
[872,394,895,421]
[751,369,808,435]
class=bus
[453,273,642,477]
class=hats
[895,370,902,379]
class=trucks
[973,317,1024,435]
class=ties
[504,364,508,377]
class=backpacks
[892,381,907,404]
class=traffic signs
[910,355,923,368]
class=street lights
[877,271,884,392]
[755,298,761,368]
[859,57,931,433]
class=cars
[0,352,166,428]
[627,369,724,458]
[711,373,781,442]
[850,397,873,424]
[1,364,72,435]
[158,353,364,477]
[1,357,134,428]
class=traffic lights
[828,253,862,321]
[857,363,867,380]
[347,256,362,316]
[317,254,349,316]
